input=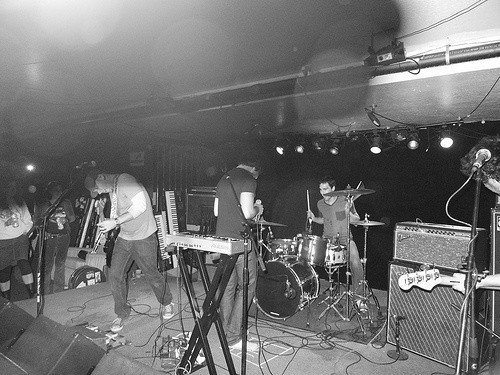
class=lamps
[438,129,454,148]
[276,138,291,156]
[406,133,419,150]
[293,134,307,154]
[369,135,383,154]
[328,137,344,156]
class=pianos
[164,187,186,268]
[152,209,173,271]
[165,231,252,375]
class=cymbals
[253,220,289,228]
[349,220,386,228]
[323,189,377,197]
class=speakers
[386,207,500,375]
[0,296,171,375]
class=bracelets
[115,218,120,226]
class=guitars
[93,192,120,269]
[397,261,500,294]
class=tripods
[308,194,385,342]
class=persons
[84,169,174,333]
[0,181,75,299]
[453,136,500,295]
[306,176,368,311]
[214,155,264,345]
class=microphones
[285,291,293,298]
[472,149,491,172]
[268,226,274,238]
[255,199,262,204]
[393,315,408,319]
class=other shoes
[111,316,129,332]
[30,291,38,298]
[355,299,372,312]
[163,303,175,319]
[227,339,260,351]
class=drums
[252,233,347,322]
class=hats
[85,169,102,198]
